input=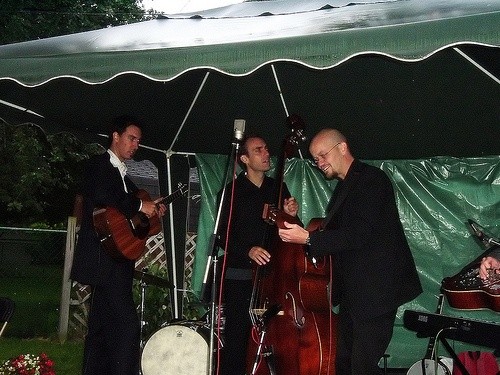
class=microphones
[255,304,282,327]
[234,120,244,142]
[469,219,491,248]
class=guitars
[93,181,192,264]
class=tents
[0,1,500,375]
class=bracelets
[306,237,310,244]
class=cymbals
[132,269,176,289]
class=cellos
[244,111,329,373]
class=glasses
[312,142,340,167]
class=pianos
[401,308,500,375]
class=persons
[479,247,500,280]
[278,129,423,375]
[213,133,299,375]
[70,118,167,375]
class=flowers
[0,352,57,375]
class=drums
[141,316,227,375]
[404,358,451,375]
